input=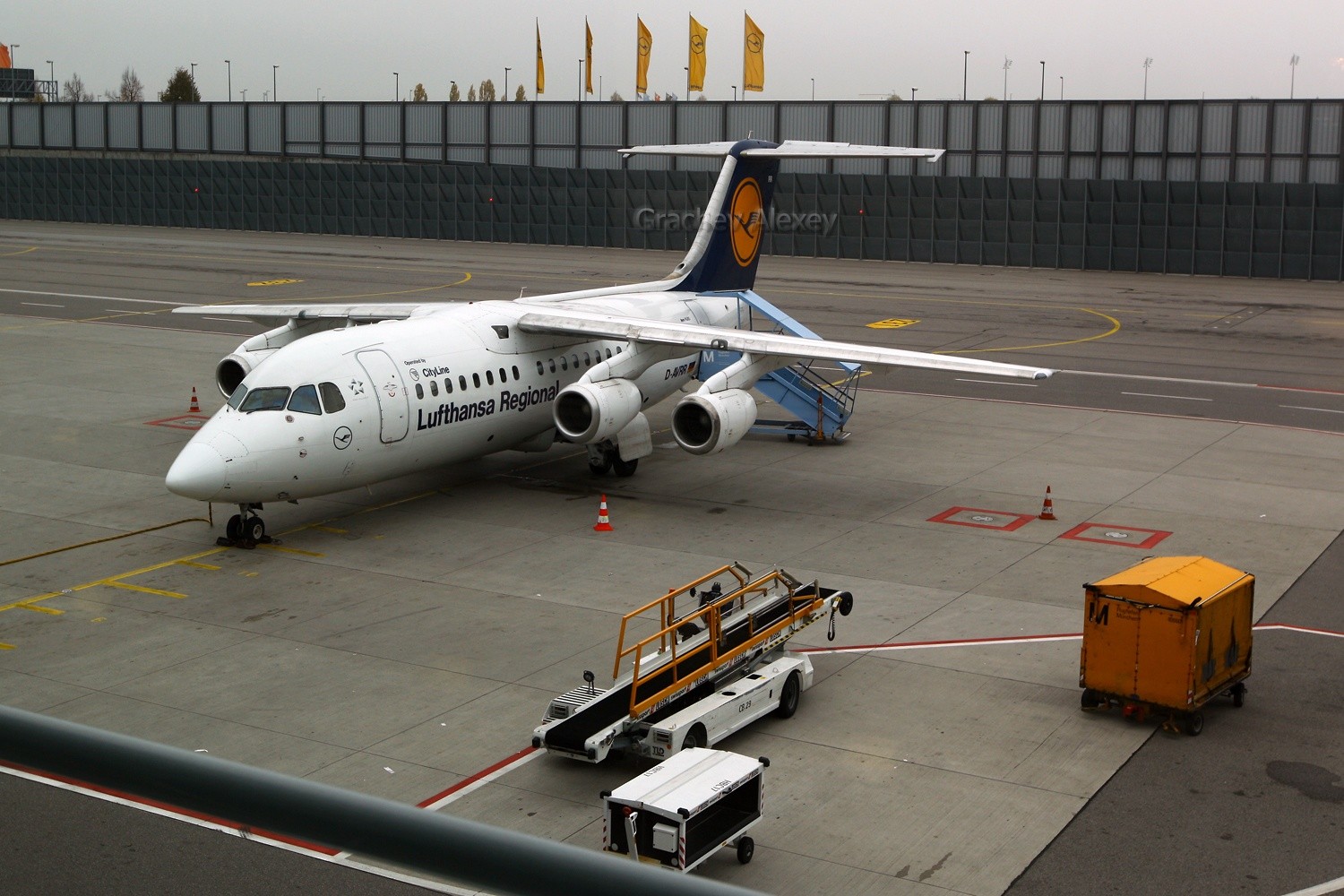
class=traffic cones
[1037,485,1058,520]
[593,493,614,531]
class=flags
[536,15,765,95]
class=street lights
[911,87,919,101]
[273,64,280,102]
[240,89,249,101]
[811,78,815,101]
[1142,56,1155,100]
[323,95,326,101]
[1060,75,1064,101]
[505,66,512,102]
[1040,61,1046,100]
[191,62,198,102]
[262,89,272,101]
[579,58,585,102]
[393,72,399,102]
[317,87,322,101]
[1289,54,1300,99]
[599,76,602,102]
[1003,59,1014,101]
[47,60,54,103]
[731,86,737,100]
[684,67,689,100]
[11,43,21,103]
[963,51,971,101]
[225,60,231,102]
[409,89,413,101]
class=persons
[671,613,698,642]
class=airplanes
[161,136,1058,551]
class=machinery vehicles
[532,558,855,771]
[600,744,771,874]
[1078,554,1257,737]
